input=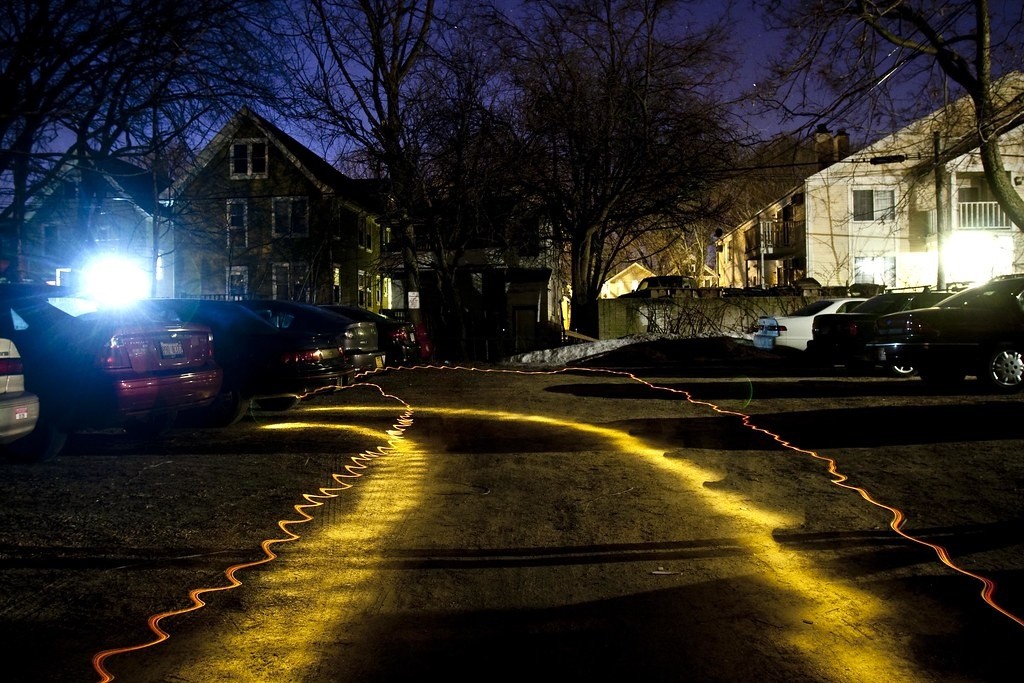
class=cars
[754,298,872,373]
[632,276,697,291]
[237,298,387,374]
[137,298,355,426]
[0,283,222,436]
[317,305,422,365]
[812,292,955,378]
[866,274,1024,394]
